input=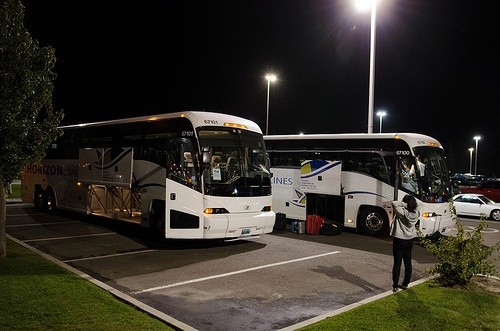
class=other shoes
[393,288,397,292]
[398,284,406,290]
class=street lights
[472,135,482,175]
[355,0,380,136]
[468,148,474,174]
[260,72,280,135]
[376,110,389,133]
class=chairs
[184,152,238,172]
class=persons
[409,157,425,192]
[390,194,420,291]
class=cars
[457,179,500,203]
[451,193,500,222]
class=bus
[18,110,277,253]
[262,131,459,241]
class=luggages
[292,215,343,236]
